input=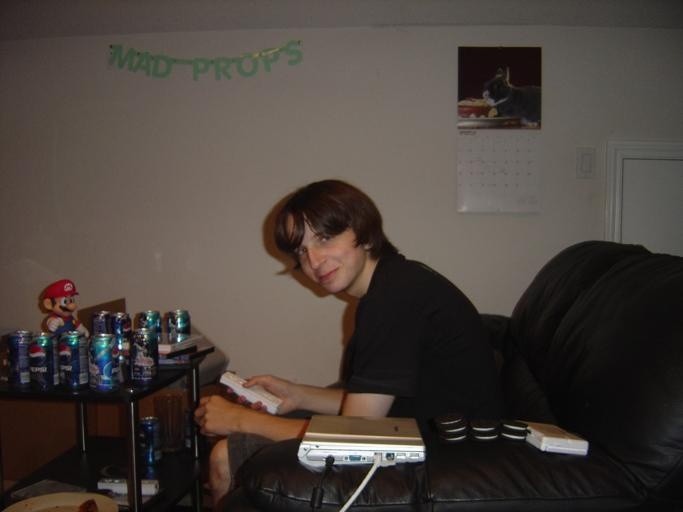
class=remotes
[219,371,284,415]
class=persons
[193,179,505,512]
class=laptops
[296,414,427,468]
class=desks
[1,347,213,510]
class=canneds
[10,310,191,391]
[136,417,164,476]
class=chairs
[215,240,683,511]
